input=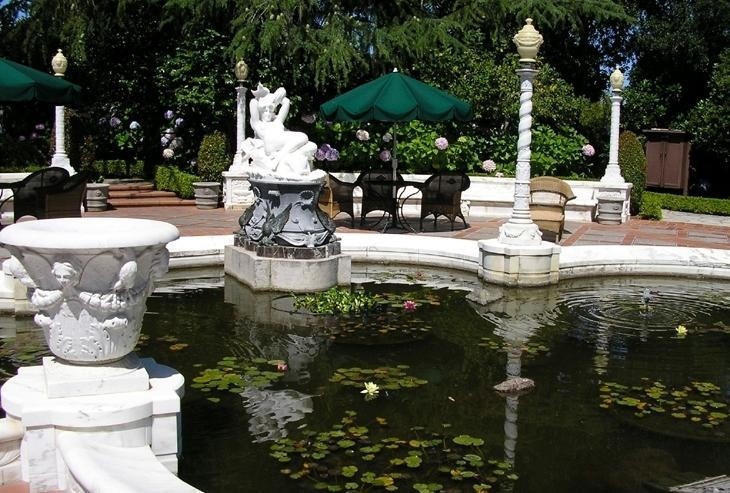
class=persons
[240,92,309,173]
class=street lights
[228,56,253,172]
[498,17,544,243]
[600,64,628,181]
[48,44,73,169]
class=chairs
[529,176,576,241]
[317,168,470,232]
[0,167,89,229]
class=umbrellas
[320,64,475,197]
[0,54,85,109]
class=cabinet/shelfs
[640,127,692,196]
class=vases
[596,196,623,226]
[192,181,221,211]
[85,182,110,212]
